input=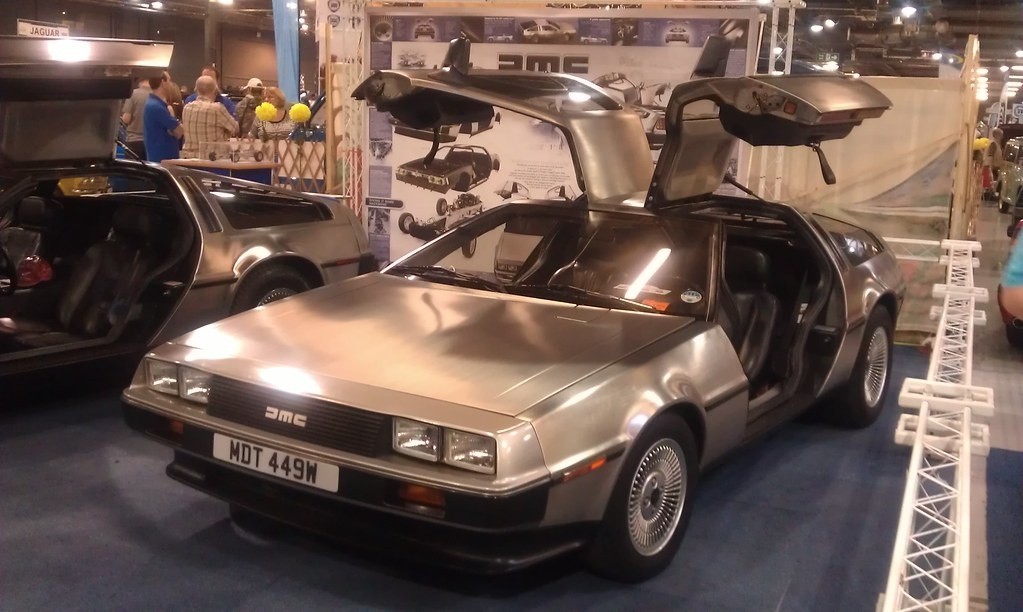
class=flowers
[290,102,311,130]
[255,102,276,130]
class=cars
[0,36,380,377]
[486,18,578,43]
[665,20,690,43]
[415,18,435,39]
[995,136,1023,214]
[592,73,669,151]
[117,31,907,587]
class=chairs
[546,228,782,380]
[0,196,55,274]
[0,203,154,352]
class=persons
[178,75,239,160]
[122,65,318,155]
[999,224,1023,322]
[248,86,298,142]
[987,126,1002,180]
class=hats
[243,78,263,90]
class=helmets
[15,255,53,287]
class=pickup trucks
[580,34,608,45]
